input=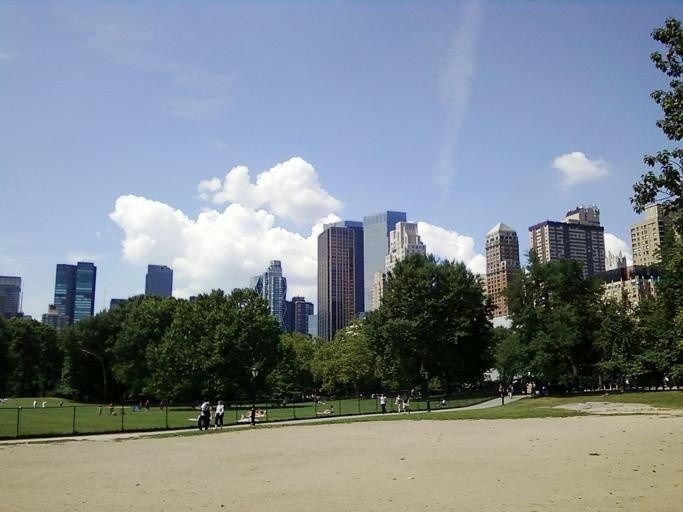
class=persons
[441,398,447,406]
[380,394,388,413]
[499,380,537,398]
[394,394,409,413]
[198,400,224,430]
